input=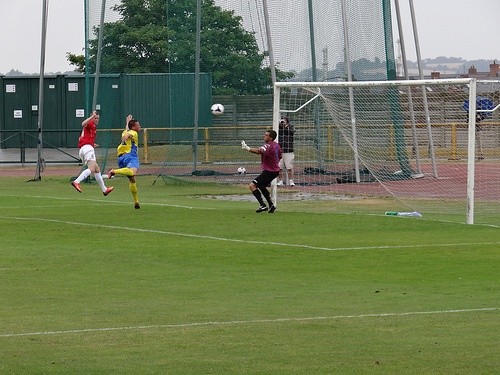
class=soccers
[238,167,246,174]
[211,103,224,115]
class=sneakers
[269,204,276,213]
[134,202,140,209]
[289,179,295,186]
[103,186,114,196]
[256,205,268,212]
[107,168,115,179]
[277,179,283,187]
[71,181,82,193]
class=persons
[241,130,282,213]
[467,108,484,157]
[277,117,295,186]
[71,111,114,196]
[108,114,141,209]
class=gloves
[240,140,250,151]
